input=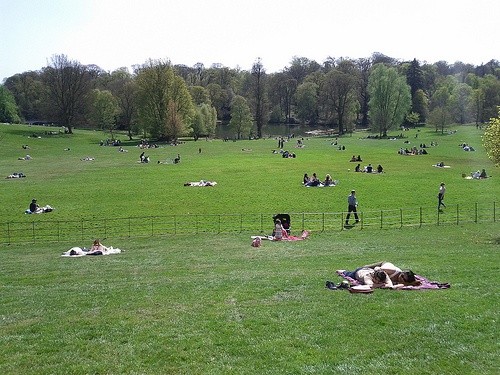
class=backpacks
[251,237,261,247]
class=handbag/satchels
[348,285,373,293]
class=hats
[375,272,386,281]
[404,270,415,283]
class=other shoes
[325,281,337,290]
[336,283,347,288]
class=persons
[6,123,489,257]
[336,260,422,289]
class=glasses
[401,274,406,282]
[375,277,381,282]
